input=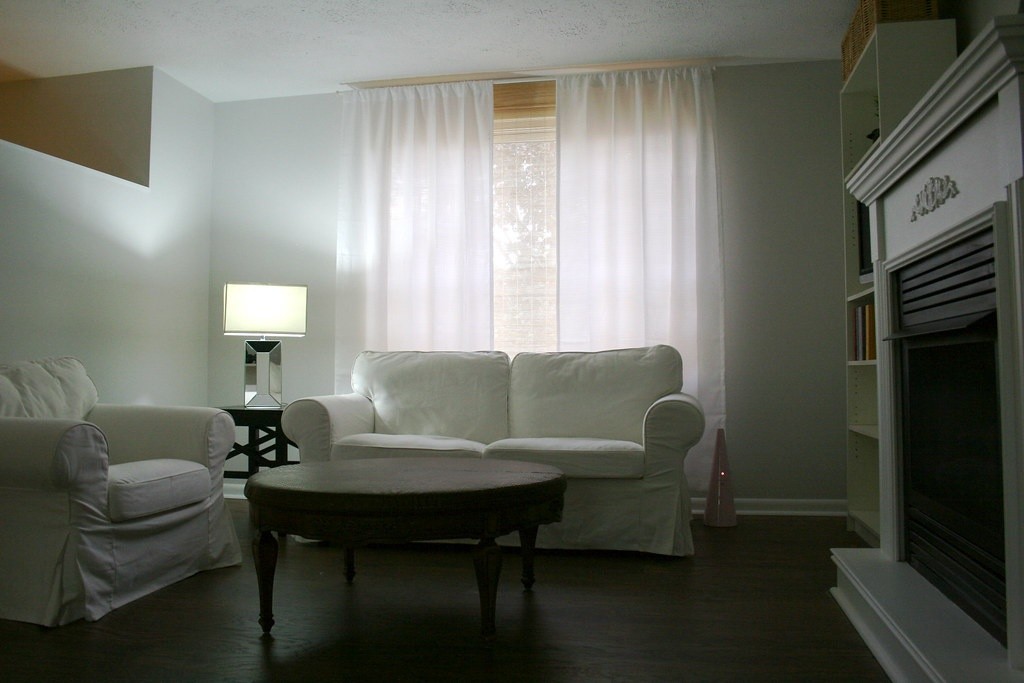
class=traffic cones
[702,429,738,527]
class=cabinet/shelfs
[838,17,958,547]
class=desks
[244,458,568,642]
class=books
[855,304,876,361]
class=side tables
[215,405,300,478]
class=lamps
[222,284,309,408]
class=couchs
[280,344,707,556]
[0,355,244,627]
